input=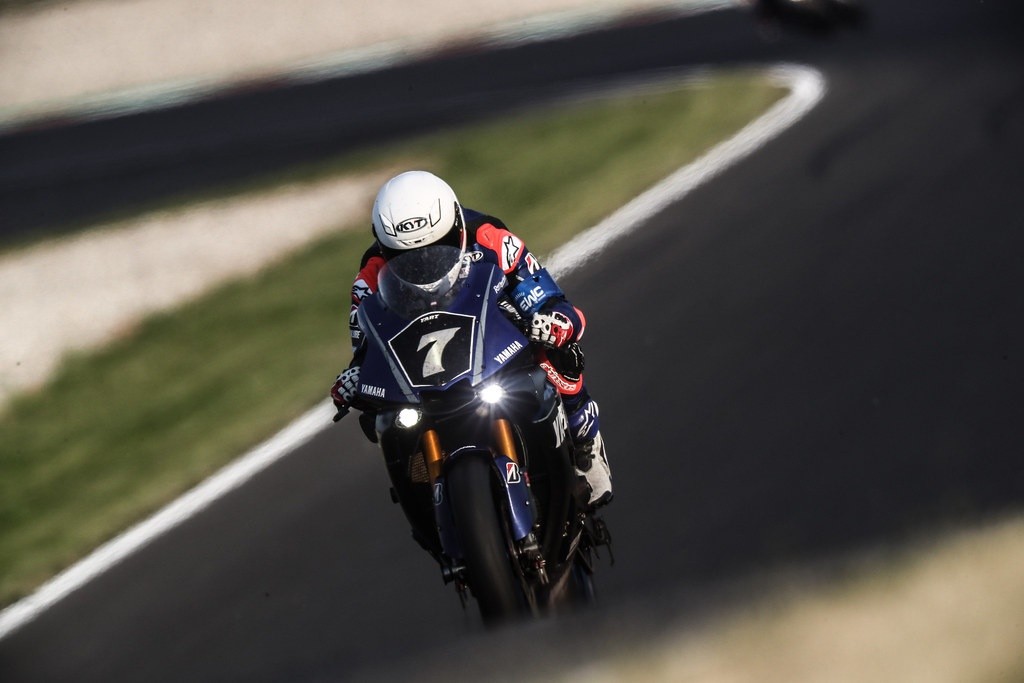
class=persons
[329,170,613,568]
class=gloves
[332,366,366,405]
[529,310,574,348]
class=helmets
[370,170,468,306]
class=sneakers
[575,430,613,506]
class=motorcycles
[332,245,616,632]
